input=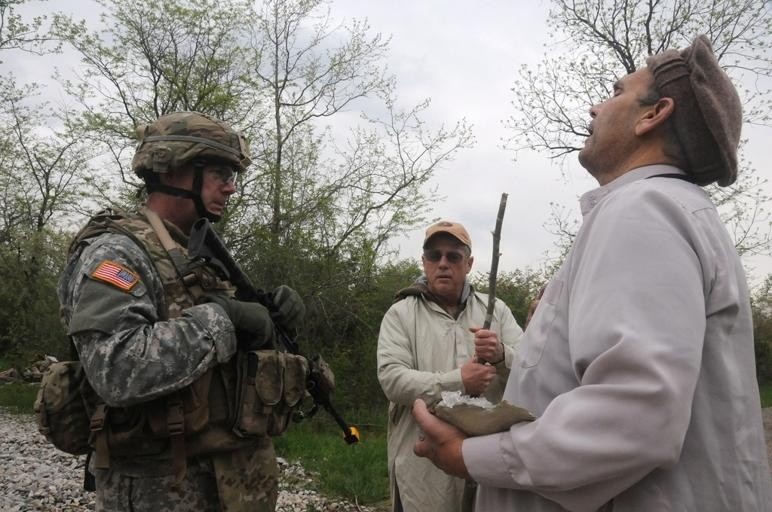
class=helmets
[130,111,252,180]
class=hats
[423,221,472,252]
[646,34,744,187]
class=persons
[375,220,524,511]
[57,109,307,511]
[410,33,771,511]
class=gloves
[270,285,306,329]
[204,294,275,349]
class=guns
[187,218,357,443]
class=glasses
[424,249,464,263]
[204,167,238,186]
